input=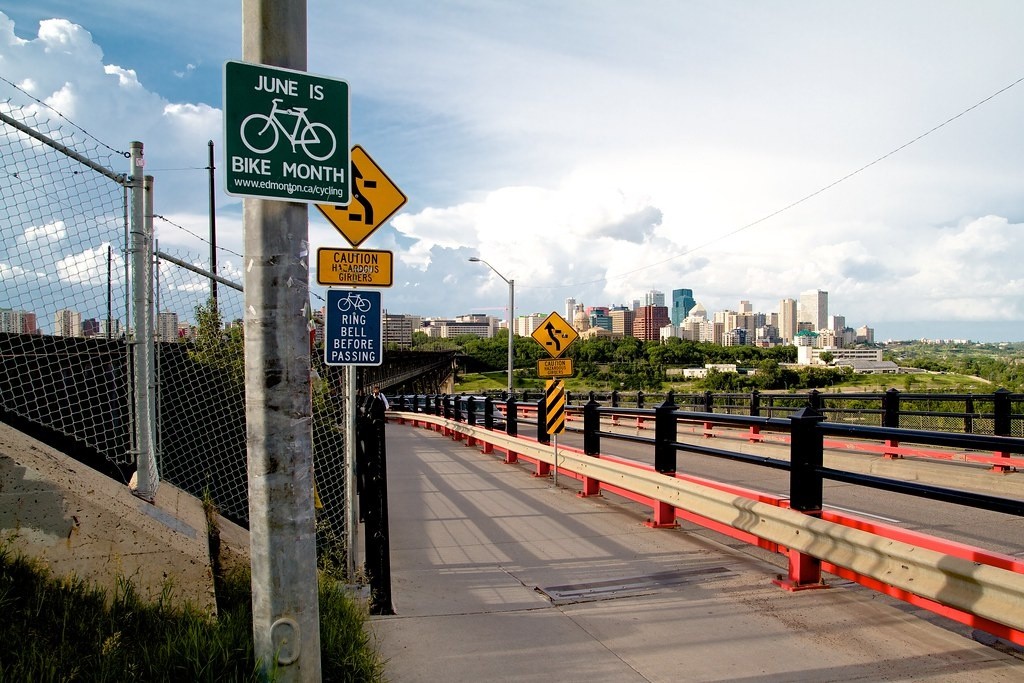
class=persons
[365,386,390,425]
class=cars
[386,394,506,431]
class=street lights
[468,256,514,401]
